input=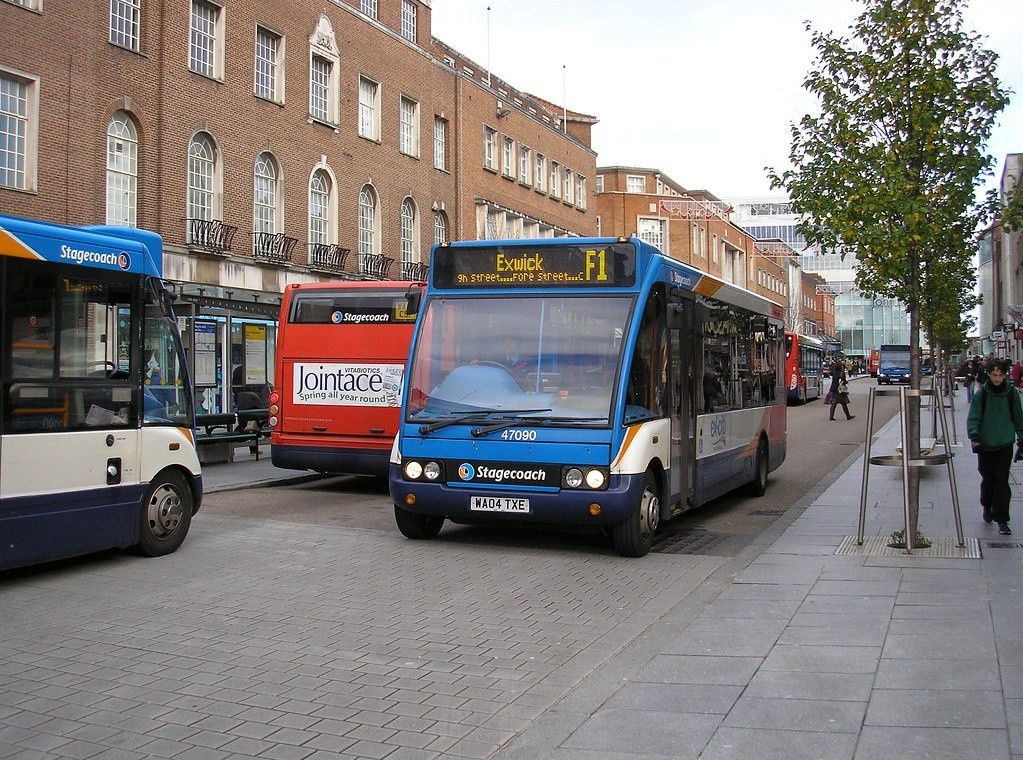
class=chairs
[235,390,270,432]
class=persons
[702,367,722,413]
[1012,360,1023,393]
[768,364,777,402]
[469,333,528,389]
[966,358,1023,534]
[964,360,974,403]
[823,363,855,421]
[954,354,1012,390]
[845,357,859,377]
[148,366,179,408]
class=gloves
[971,439,982,453]
[1014,446,1023,463]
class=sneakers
[983,507,993,523]
[998,522,1012,534]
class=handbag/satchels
[1013,380,1020,387]
[963,379,968,387]
[838,379,849,394]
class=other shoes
[830,418,836,421]
[847,415,855,420]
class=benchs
[894,438,937,456]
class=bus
[785,332,824,404]
[268,281,455,476]
[0,213,203,572]
[877,344,922,385]
[389,236,787,558]
[922,358,937,375]
[870,349,879,378]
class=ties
[507,359,513,367]
